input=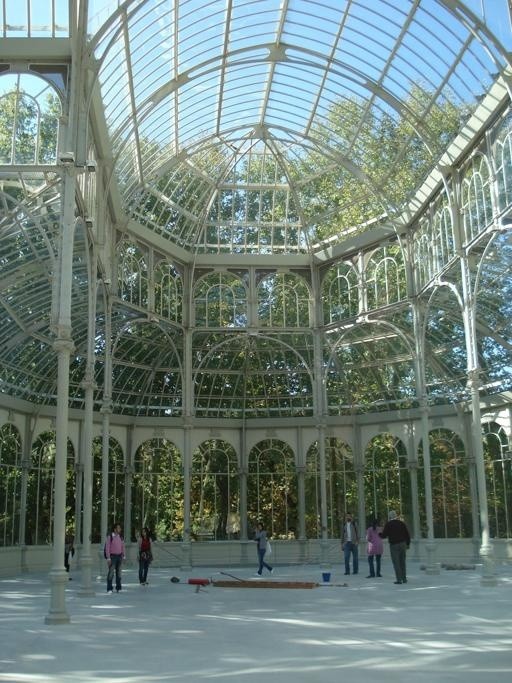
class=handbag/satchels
[264,540,272,557]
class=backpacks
[104,533,123,559]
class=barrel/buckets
[322,573,331,582]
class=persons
[103,523,125,594]
[378,510,410,584]
[64,541,74,573]
[340,513,360,575]
[138,527,152,586]
[366,519,384,577]
[253,522,275,575]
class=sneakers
[106,581,148,594]
[343,571,408,584]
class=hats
[389,511,400,520]
